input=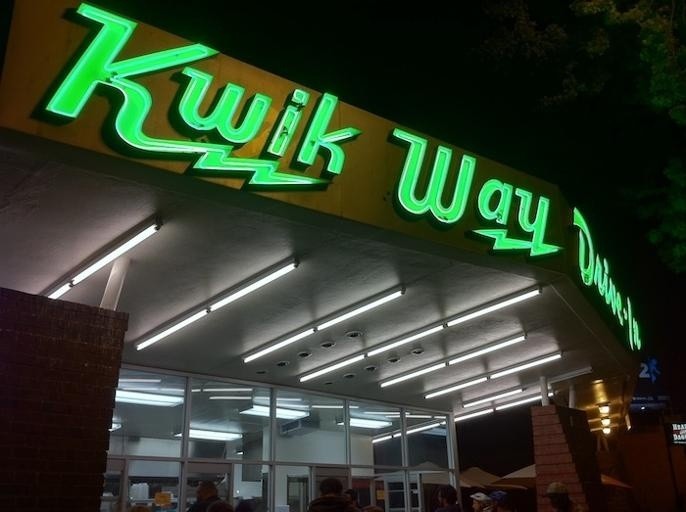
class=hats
[538,482,569,498]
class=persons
[489,490,511,512]
[344,489,362,509]
[469,492,494,512]
[543,483,584,512]
[207,500,233,512]
[434,485,461,512]
[361,504,384,512]
[236,497,267,512]
[187,480,222,512]
[309,479,361,512]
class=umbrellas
[483,463,633,488]
[373,461,485,488]
[458,466,527,489]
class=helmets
[469,492,491,503]
[489,490,508,504]
